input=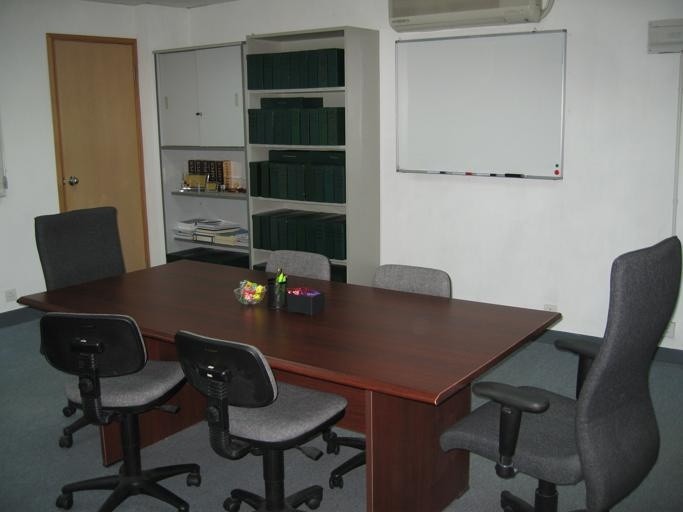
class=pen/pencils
[269,268,287,282]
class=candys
[237,278,265,305]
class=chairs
[38,311,204,511]
[264,250,331,282]
[437,236,681,511]
[175,327,350,512]
[327,264,450,491]
[32,206,127,449]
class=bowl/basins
[184,172,210,192]
[233,287,267,306]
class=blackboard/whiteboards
[395,29,567,180]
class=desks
[13,260,563,509]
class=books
[171,158,248,248]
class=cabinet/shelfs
[244,27,379,286]
[153,42,251,270]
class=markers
[428,171,525,178]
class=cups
[270,277,286,309]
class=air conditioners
[388,0,542,32]
